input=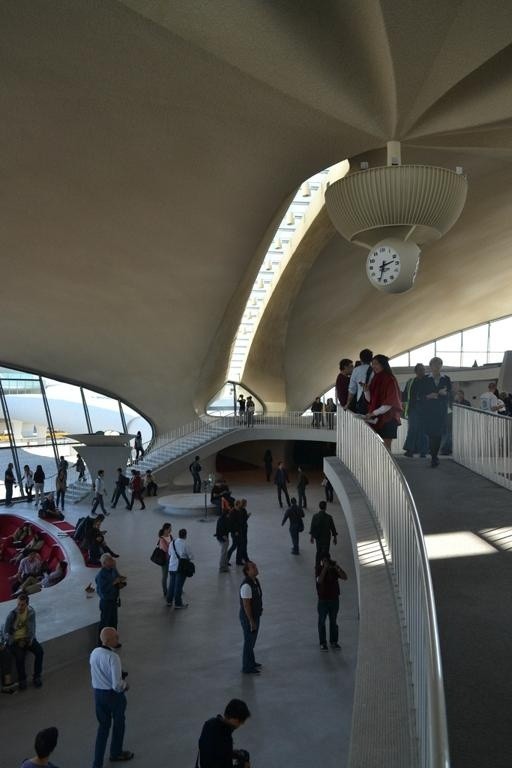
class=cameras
[327,561,337,565]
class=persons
[22,726,57,768]
[311,397,337,429]
[1,433,347,693]
[237,395,255,424]
[199,699,251,768]
[335,349,512,468]
[89,627,134,768]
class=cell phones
[358,381,365,386]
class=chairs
[0,514,64,603]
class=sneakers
[249,663,261,673]
[110,750,134,761]
[319,644,341,652]
[167,602,187,609]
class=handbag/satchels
[151,547,166,565]
[178,558,195,577]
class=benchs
[42,517,106,569]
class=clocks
[365,238,421,294]
